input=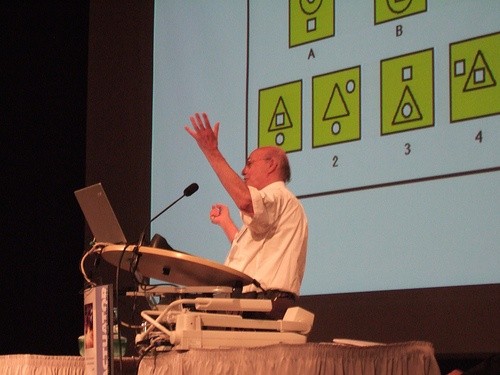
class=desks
[138,342,440,375]
[0,353,139,375]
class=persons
[184,111,308,321]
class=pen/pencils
[208,208,219,223]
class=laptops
[74,183,127,245]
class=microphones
[138,183,199,247]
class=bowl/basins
[77,336,127,357]
